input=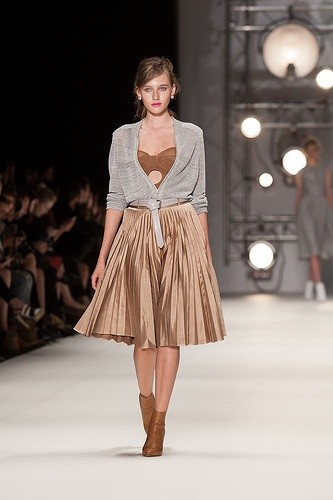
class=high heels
[12,304,45,329]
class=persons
[72,57,226,457]
[0,163,105,363]
[295,136,333,300]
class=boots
[139,393,155,434]
[143,411,166,457]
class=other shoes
[47,314,64,329]
[316,282,326,300]
[305,281,314,299]
[61,303,86,315]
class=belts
[129,198,189,248]
